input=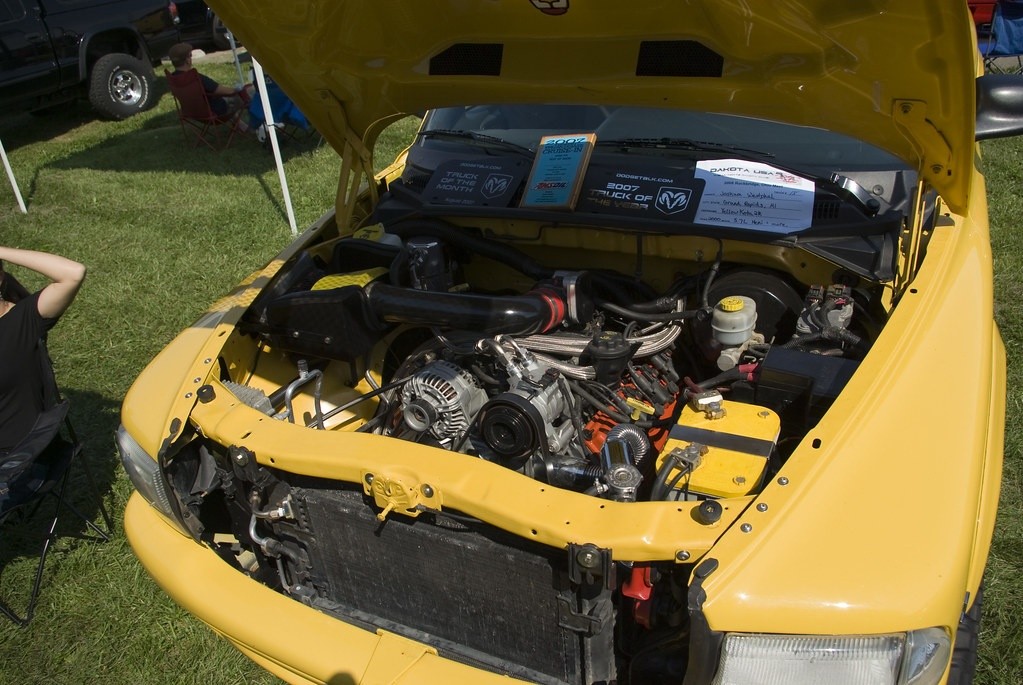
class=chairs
[967,0,1023,75]
[0,339,114,625]
[164,68,327,156]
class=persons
[169,42,248,133]
[0,246,86,519]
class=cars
[174,0,243,52]
[115,0,1022,685]
[0,0,182,121]
